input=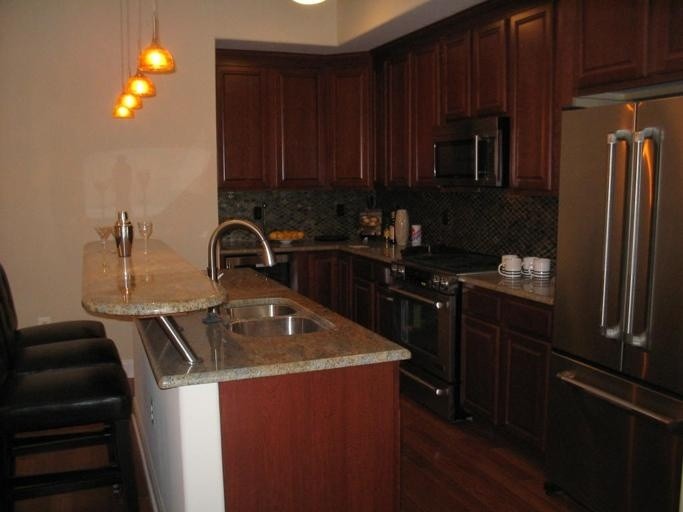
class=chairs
[0,261,140,512]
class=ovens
[386,279,458,421]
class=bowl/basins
[280,241,291,245]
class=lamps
[113,0,177,120]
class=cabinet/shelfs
[386,39,438,189]
[437,8,507,117]
[338,254,381,333]
[292,252,339,314]
[370,47,390,190]
[215,48,327,192]
[461,282,554,458]
[507,1,553,197]
[556,0,683,103]
[328,51,371,192]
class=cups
[409,224,422,246]
[497,254,550,280]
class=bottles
[383,223,388,242]
[117,255,135,305]
[388,209,396,244]
[111,210,134,257]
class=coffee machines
[357,208,385,242]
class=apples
[270,230,304,239]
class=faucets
[209,217,277,316]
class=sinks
[227,304,298,319]
[230,316,319,337]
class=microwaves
[430,113,510,190]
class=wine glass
[135,220,153,255]
[93,225,115,254]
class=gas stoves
[391,245,498,295]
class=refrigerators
[542,94,682,512]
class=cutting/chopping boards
[313,235,349,241]
